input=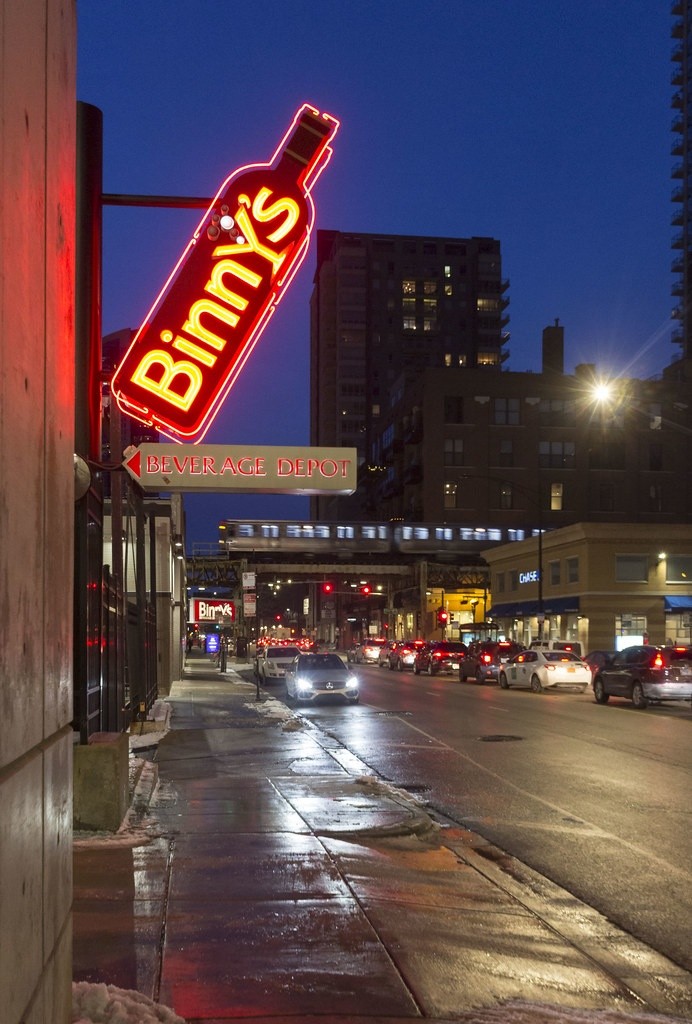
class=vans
[529,640,585,661]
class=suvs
[388,640,435,671]
[412,642,468,676]
[355,638,385,664]
[457,642,516,683]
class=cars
[498,650,591,693]
[346,643,361,662]
[285,653,359,705]
[378,641,403,670]
[584,650,622,684]
[592,644,692,709]
[257,636,316,651]
[257,646,302,686]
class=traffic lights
[324,584,332,591]
[449,613,455,625]
[277,616,280,620]
[214,624,220,631]
[363,587,369,593]
[193,624,198,629]
[439,610,447,628]
